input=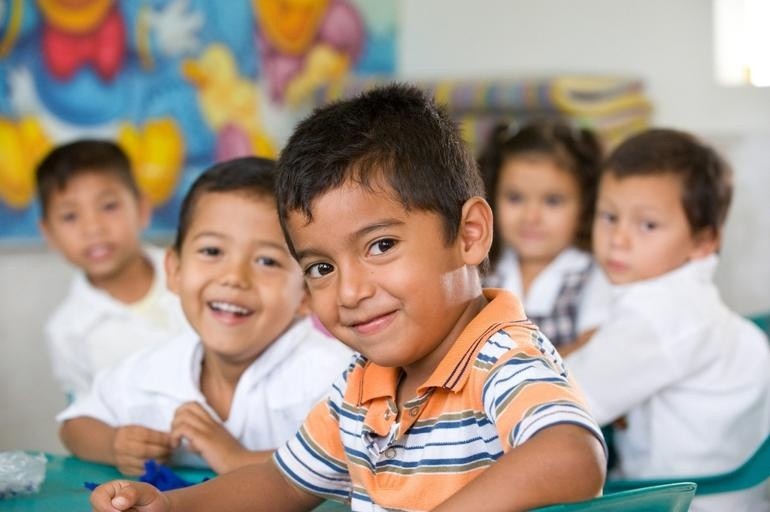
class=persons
[61,158,366,483]
[87,85,609,512]
[480,117,611,362]
[37,141,188,408]
[552,129,770,500]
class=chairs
[602,311,770,512]
[527,481,698,512]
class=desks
[0,448,348,512]
